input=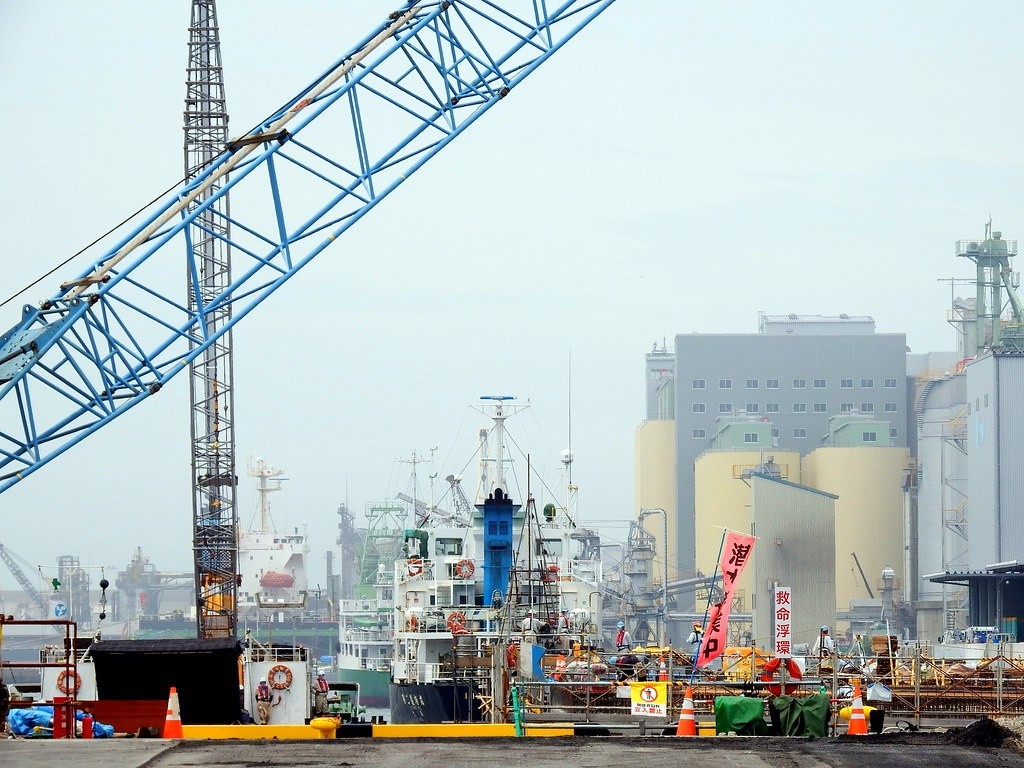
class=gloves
[315,692,319,696]
[256,694,258,702]
[269,696,273,703]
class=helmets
[821,626,828,630]
[318,671,325,676]
[260,678,266,683]
[528,610,537,614]
[560,613,563,617]
[240,685,244,690]
[617,622,624,627]
[694,621,702,626]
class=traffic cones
[161,687,185,740]
[846,685,870,736]
[676,687,697,737]
[657,655,668,682]
[555,654,569,681]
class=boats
[132,457,340,665]
[387,396,578,725]
[336,497,411,708]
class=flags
[699,534,756,668]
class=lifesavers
[448,613,467,631]
[408,612,417,632]
[57,670,81,694]
[267,665,292,690]
[507,644,518,667]
[544,566,561,582]
[456,559,475,578]
[761,658,801,697]
[407,558,423,577]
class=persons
[611,655,638,686]
[838,659,854,684]
[255,678,274,725]
[813,626,832,672]
[311,671,329,716]
[556,609,570,654]
[522,609,546,644]
[0,677,10,732]
[686,620,705,663]
[615,622,632,656]
